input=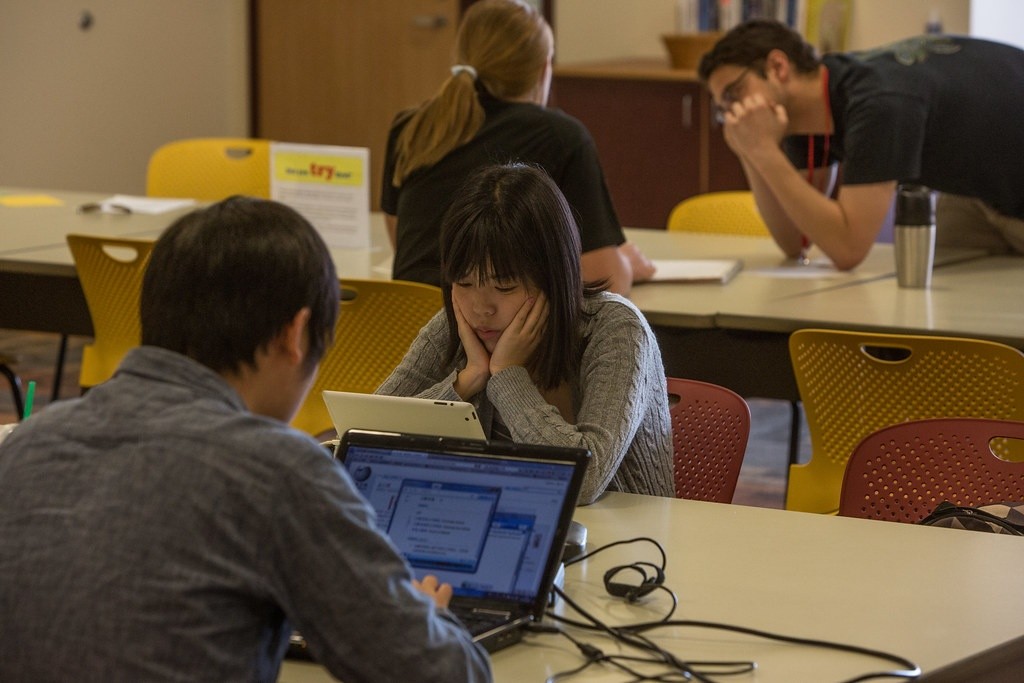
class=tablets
[324,390,486,442]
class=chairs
[782,325,1024,516]
[834,419,1024,536]
[665,376,752,506]
[288,278,445,438]
[66,232,160,397]
[666,189,777,239]
[145,137,273,206]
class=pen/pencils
[22,380,36,421]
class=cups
[895,185,936,290]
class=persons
[369,163,677,507]
[696,16,1024,271]
[1,195,494,683]
[379,0,655,295]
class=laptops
[282,427,592,659]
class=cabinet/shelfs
[552,52,795,230]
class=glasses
[713,67,753,123]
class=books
[94,193,193,216]
[633,259,743,286]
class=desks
[717,254,1024,508]
[0,186,216,408]
[0,419,1024,683]
[0,211,1024,463]
